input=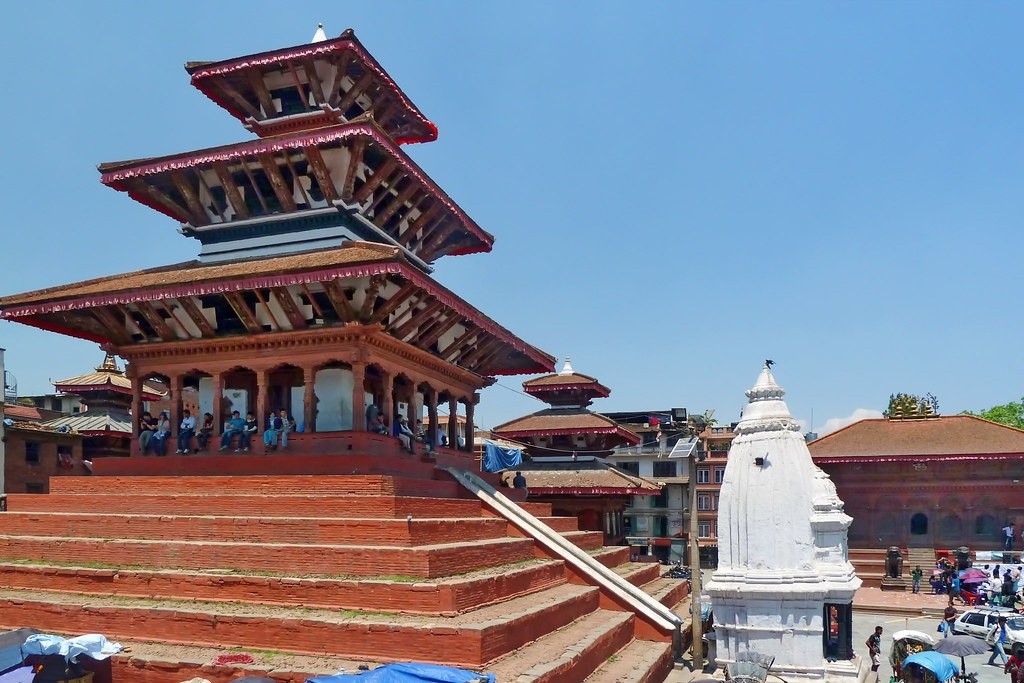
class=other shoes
[404,447,411,451]
[219,446,229,451]
[234,448,242,452]
[176,449,183,454]
[430,451,440,455]
[243,447,249,451]
[183,449,189,454]
[270,445,277,450]
[279,447,287,452]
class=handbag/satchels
[1007,663,1018,673]
[938,623,944,632]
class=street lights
[668,436,703,669]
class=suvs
[952,606,1024,656]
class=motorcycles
[662,560,704,593]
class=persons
[369,412,390,434]
[909,565,924,593]
[1001,522,1015,550]
[941,601,957,638]
[139,411,158,453]
[985,616,1015,666]
[866,626,883,681]
[176,409,197,455]
[498,472,510,487]
[1004,641,1024,683]
[152,411,171,456]
[436,424,448,446]
[457,433,464,448]
[513,471,526,488]
[235,412,258,452]
[417,418,439,455]
[270,408,295,450]
[393,414,418,454]
[948,573,967,606]
[218,410,247,450]
[197,412,214,451]
[929,557,1024,612]
[263,409,281,450]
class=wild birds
[766,359,777,370]
